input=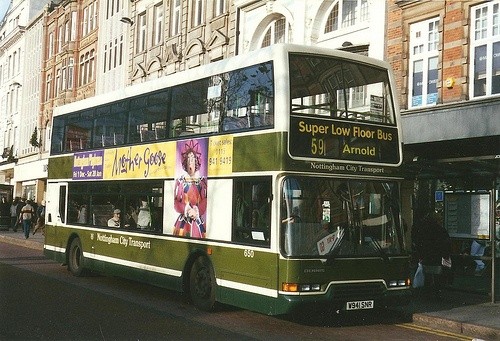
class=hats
[114,209,121,213]
[181,140,203,172]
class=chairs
[57,107,273,152]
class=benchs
[93,210,125,229]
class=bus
[43,43,412,316]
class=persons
[174,139,207,239]
[79,200,299,238]
[1,197,46,239]
[411,202,500,301]
